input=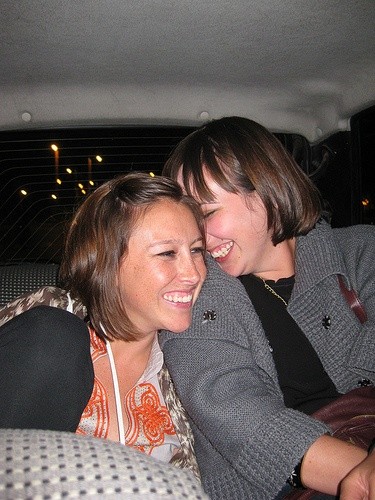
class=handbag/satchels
[279,385,375,499]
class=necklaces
[254,273,289,307]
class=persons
[1,172,207,488]
[158,114,374,500]
